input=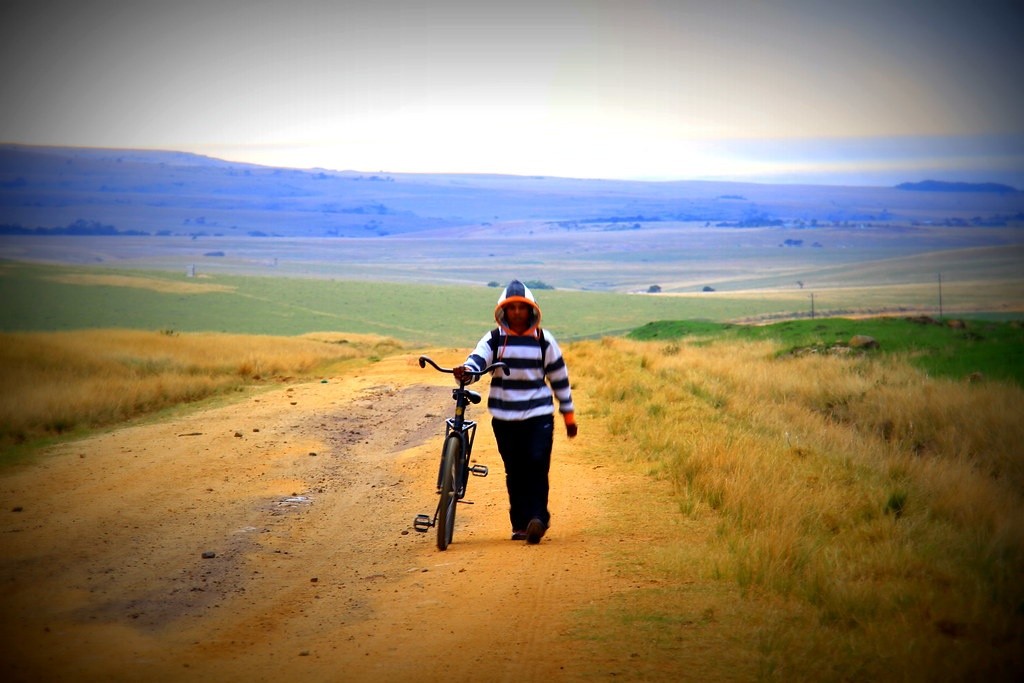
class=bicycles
[413,356,510,552]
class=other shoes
[526,518,542,544]
[512,529,527,540]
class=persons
[453,280,578,545]
